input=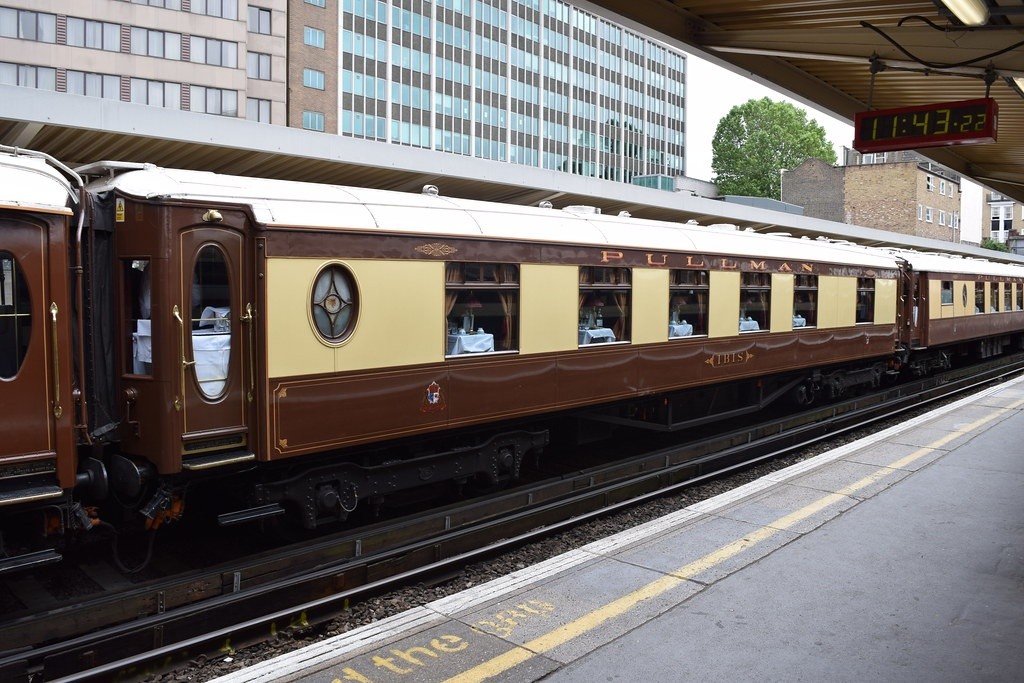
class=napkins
[200,306,230,327]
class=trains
[0,144,1024,578]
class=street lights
[780,168,789,203]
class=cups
[459,329,466,336]
[477,328,484,335]
[452,322,457,334]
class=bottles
[596,309,603,328]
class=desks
[578,327,616,345]
[668,325,693,336]
[448,333,494,355]
[133,330,231,395]
[739,321,760,331]
[793,318,806,327]
